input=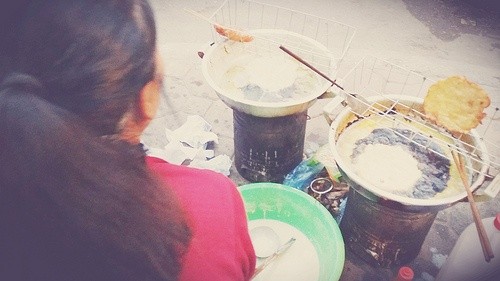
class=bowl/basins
[248,227,279,259]
[237,182,345,281]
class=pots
[328,95,499,214]
[195,29,339,118]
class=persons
[0,1,256,280]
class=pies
[424,76,492,135]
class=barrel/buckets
[233,108,309,183]
[339,187,438,267]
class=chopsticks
[447,144,494,264]
[249,238,295,281]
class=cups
[307,178,333,211]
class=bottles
[436,213,500,281]
[392,267,415,280]
